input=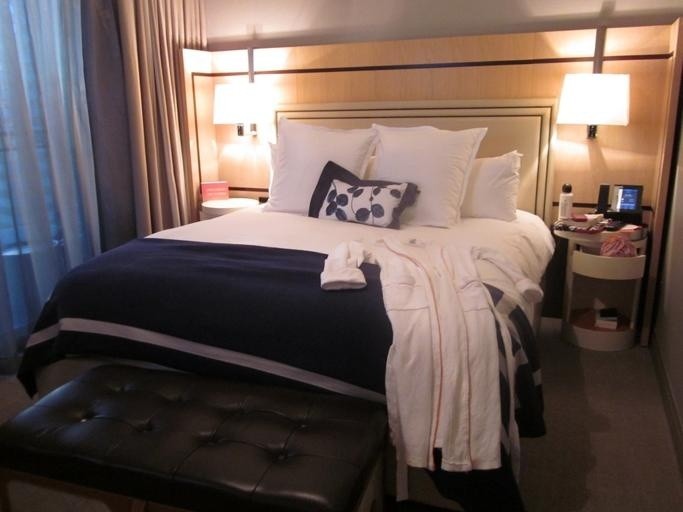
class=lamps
[553,72,629,140]
[213,81,274,140]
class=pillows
[459,152,524,224]
[364,123,490,228]
[304,161,420,230]
[262,112,381,221]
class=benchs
[2,364,385,511]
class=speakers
[597,184,610,211]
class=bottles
[597,184,611,213]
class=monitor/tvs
[610,184,644,210]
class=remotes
[606,219,622,231]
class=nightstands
[556,222,646,353]
[200,197,260,222]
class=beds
[15,98,553,508]
